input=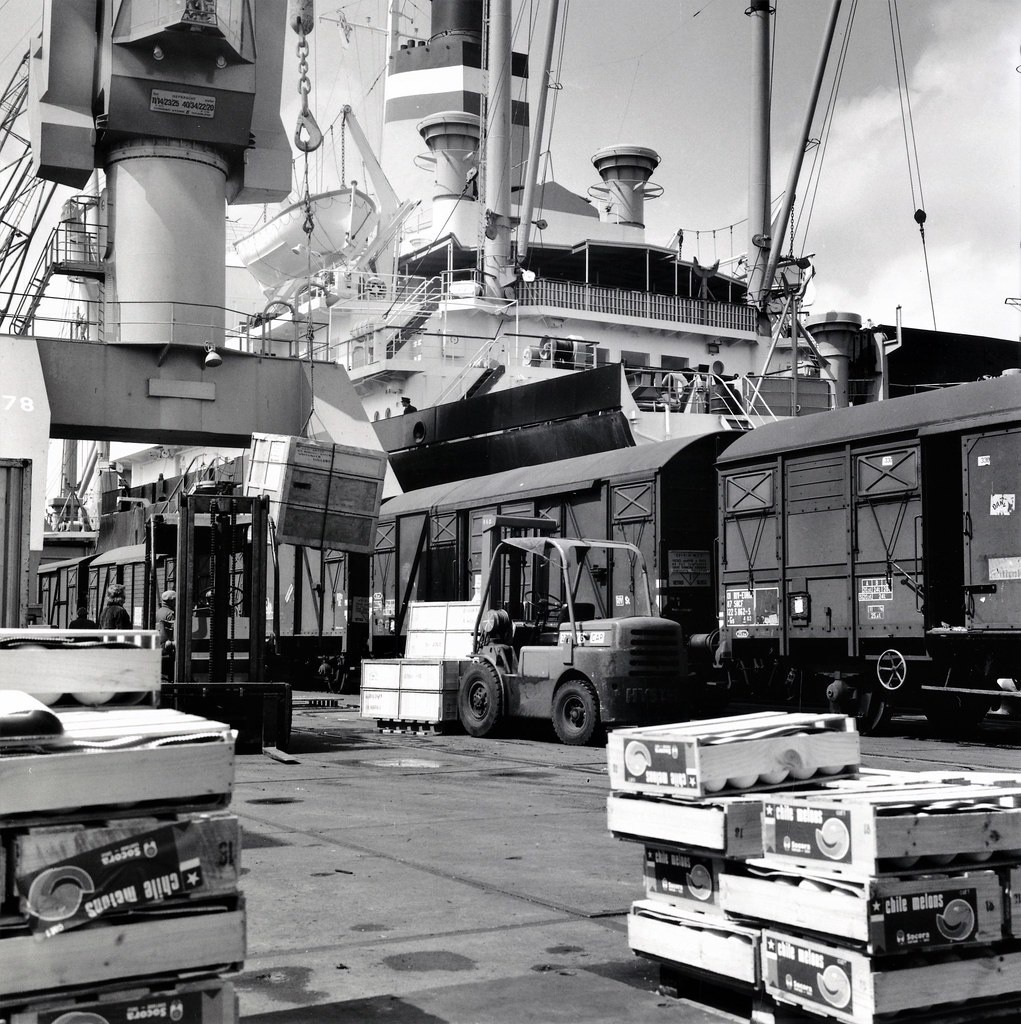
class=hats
[161,590,177,603]
[76,607,89,616]
[107,584,125,598]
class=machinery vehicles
[372,514,689,744]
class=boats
[233,188,377,287]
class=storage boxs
[356,601,483,722]
[240,432,389,558]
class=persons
[99,584,133,629]
[400,397,417,415]
[156,590,176,623]
[65,607,99,642]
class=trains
[32,360,1020,749]
